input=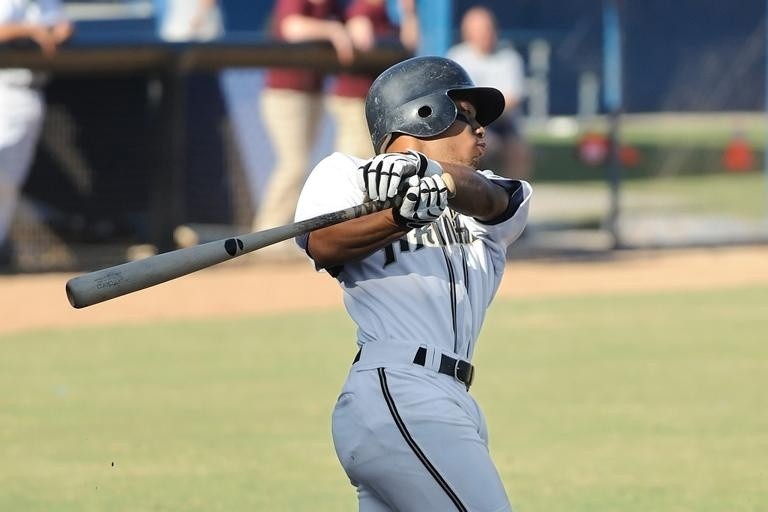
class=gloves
[357,150,444,202]
[391,174,448,229]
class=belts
[352,345,475,393]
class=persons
[292,55,534,511]
[1,0,75,252]
[321,0,422,160]
[250,1,356,261]
[154,0,231,251]
[440,7,527,238]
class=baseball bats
[64,172,459,308]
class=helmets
[365,57,505,155]
